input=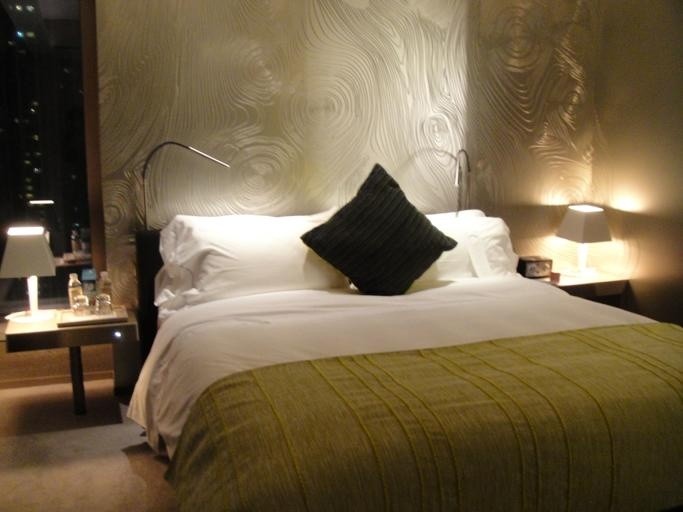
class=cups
[72,294,114,316]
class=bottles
[69,229,80,256]
[67,270,111,306]
[80,233,90,254]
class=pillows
[299,161,457,298]
[432,209,485,219]
[153,204,349,313]
[403,215,518,291]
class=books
[56,304,129,327]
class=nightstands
[5,305,142,415]
[532,274,628,300]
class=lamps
[133,140,230,232]
[556,199,614,281]
[0,225,57,324]
[454,149,474,210]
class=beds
[125,209,682,512]
